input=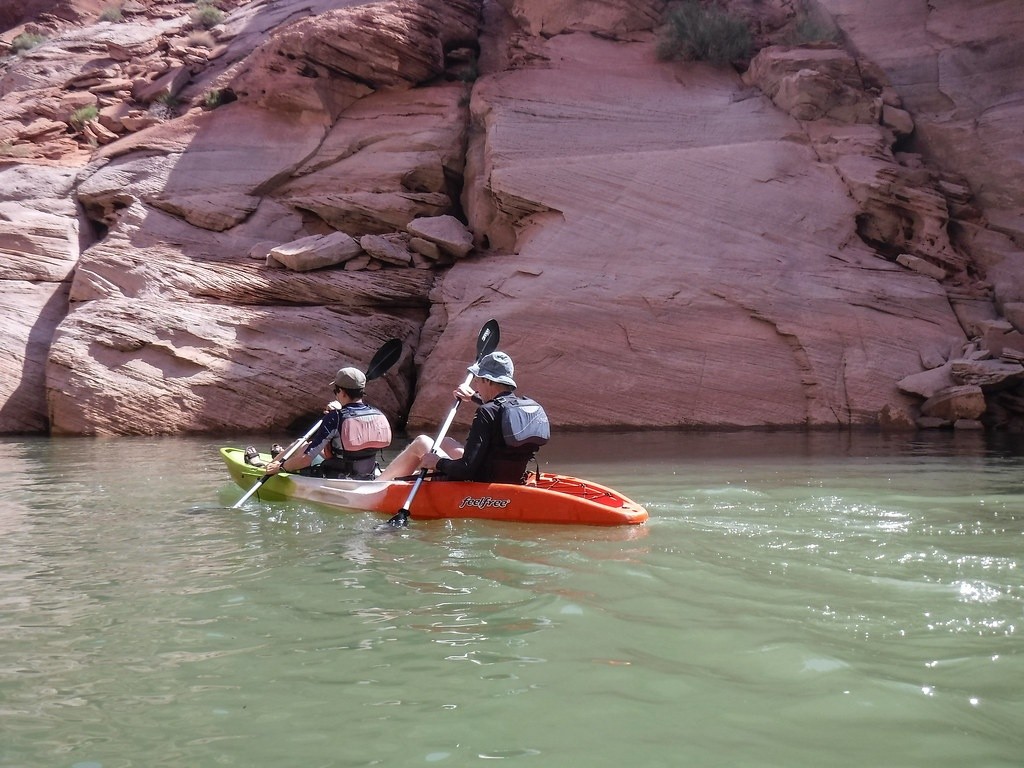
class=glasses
[333,389,340,395]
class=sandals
[244,445,265,468]
[271,443,285,460]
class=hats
[466,351,517,391]
[329,367,366,389]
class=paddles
[233,339,403,510]
[387,318,500,529]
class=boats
[219,447,648,527]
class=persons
[373,351,537,484]
[244,367,377,480]
